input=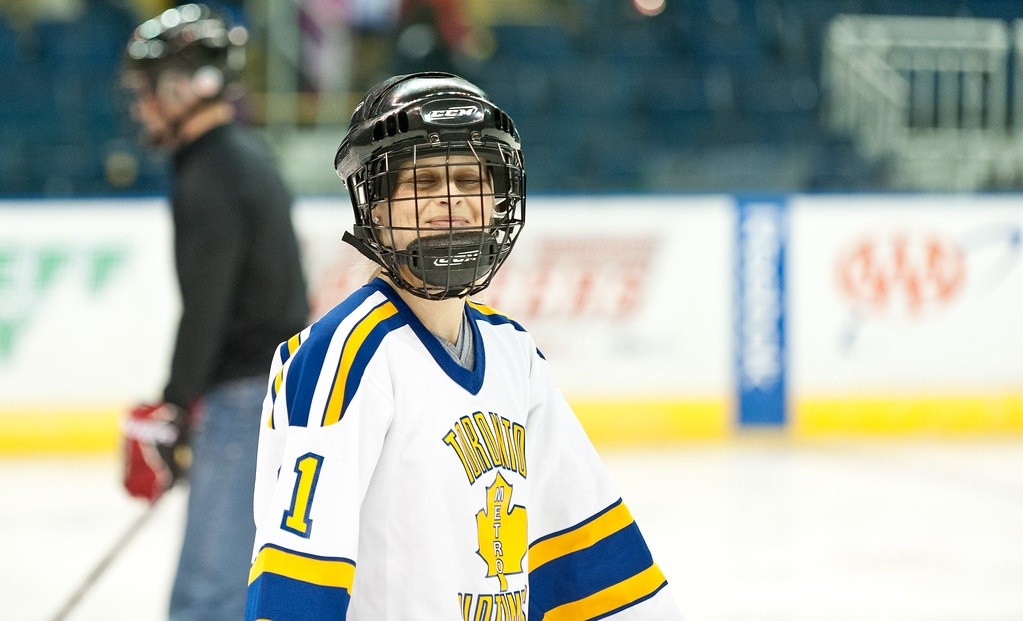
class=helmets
[335,71,528,300]
[119,5,248,101]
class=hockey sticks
[53,483,173,621]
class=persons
[115,3,314,621]
[243,71,684,621]
